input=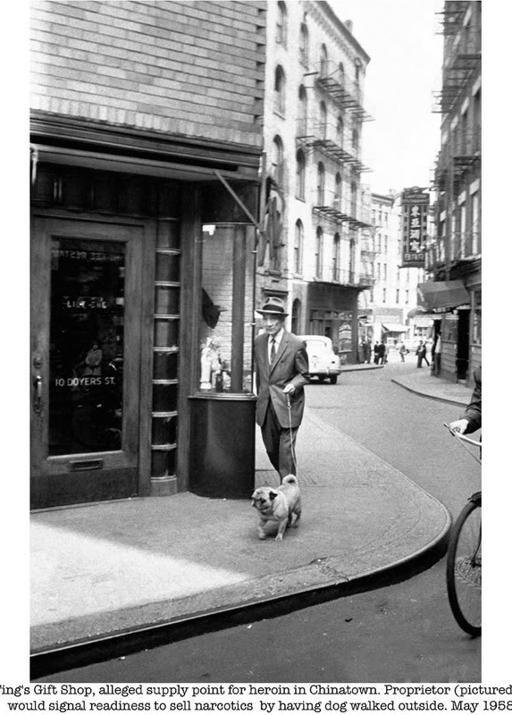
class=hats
[255,296,290,319]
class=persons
[360,337,430,369]
[249,296,311,486]
[447,364,481,438]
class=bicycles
[443,419,481,638]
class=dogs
[248,472,303,542]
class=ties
[269,338,278,365]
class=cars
[299,335,342,385]
